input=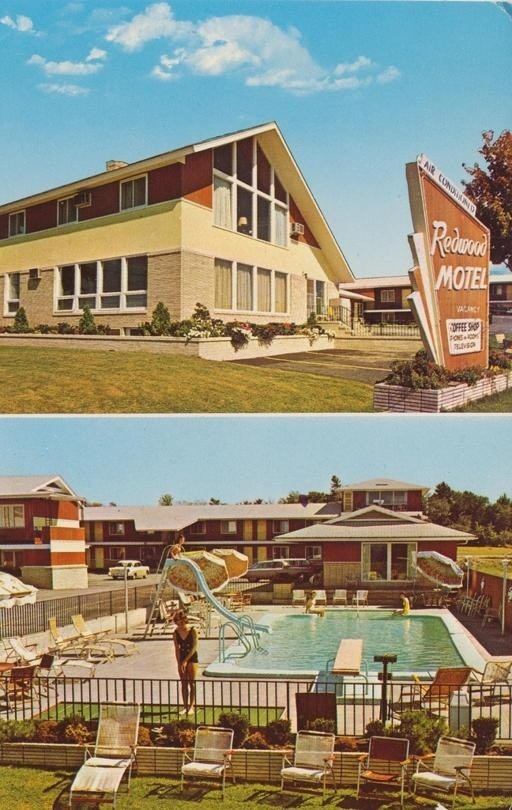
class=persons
[305,591,326,619]
[166,534,186,561]
[391,592,410,617]
[171,612,200,716]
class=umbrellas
[208,548,249,583]
[167,549,229,603]
[412,550,465,590]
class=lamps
[302,271,307,278]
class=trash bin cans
[447,691,472,737]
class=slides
[165,555,273,634]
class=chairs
[356,660,512,810]
[333,588,347,607]
[159,590,252,636]
[312,590,327,607]
[457,590,502,629]
[0,615,141,810]
[352,589,368,608]
[281,730,337,806]
[292,590,307,607]
[181,726,236,801]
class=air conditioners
[290,222,305,236]
[29,267,42,279]
[73,191,92,208]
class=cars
[108,560,150,580]
[238,560,314,582]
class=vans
[273,558,318,581]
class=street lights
[373,651,398,727]
[117,560,134,634]
[464,553,473,599]
[500,558,511,636]
